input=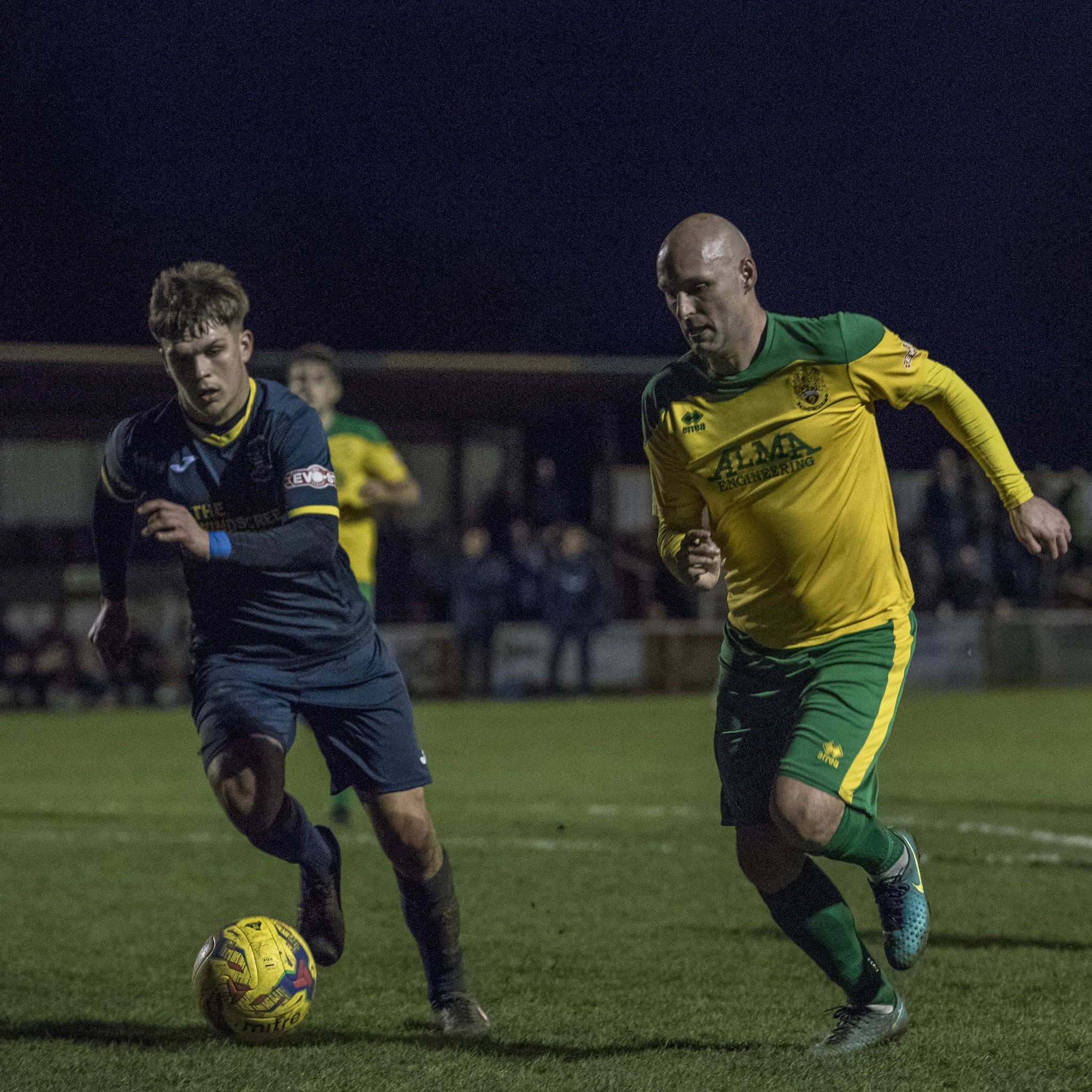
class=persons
[641,214,1071,1057]
[288,348,424,824]
[916,451,1070,623]
[89,259,496,1042]
[439,457,616,695]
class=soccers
[191,917,317,1044]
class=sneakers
[808,989,910,1057]
[431,990,493,1041]
[866,827,933,969]
[294,826,345,966]
